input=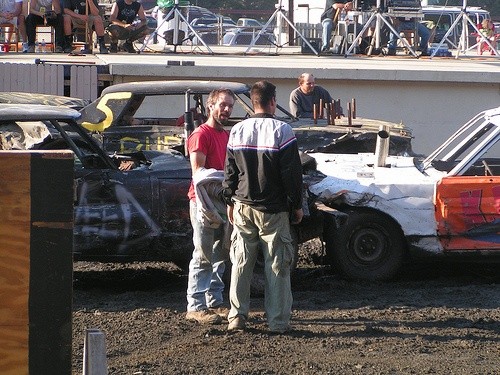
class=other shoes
[27,46,35,53]
[55,45,63,53]
[109,43,118,53]
[210,306,229,319]
[186,308,221,326]
[228,315,246,332]
[123,41,136,53]
[22,42,28,53]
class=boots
[99,39,108,53]
[64,35,73,53]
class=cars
[288,108,500,284]
[0,79,414,271]
[142,2,277,46]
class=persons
[106,0,148,53]
[24,0,65,53]
[220,82,302,333]
[60,0,110,54]
[383,0,429,56]
[289,73,346,118]
[185,87,235,324]
[321,0,362,52]
[0,0,29,53]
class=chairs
[471,24,500,55]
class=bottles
[341,7,346,21]
[35,39,39,53]
[41,39,46,53]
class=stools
[71,24,93,51]
[35,25,54,52]
[0,23,19,52]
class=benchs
[371,27,415,53]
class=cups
[40,6,45,13]
